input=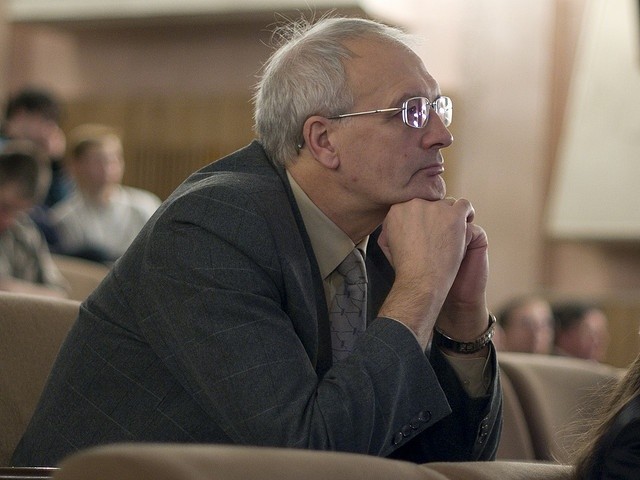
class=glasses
[296,97,454,156]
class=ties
[329,250,368,364]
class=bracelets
[430,310,496,355]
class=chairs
[0,291,81,467]
[55,255,110,303]
[500,351,627,459]
[496,371,536,459]
[49,442,449,479]
[426,458,575,479]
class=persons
[0,89,81,256]
[552,300,611,368]
[48,119,163,266]
[5,6,502,468]
[497,292,557,360]
[0,155,69,299]
